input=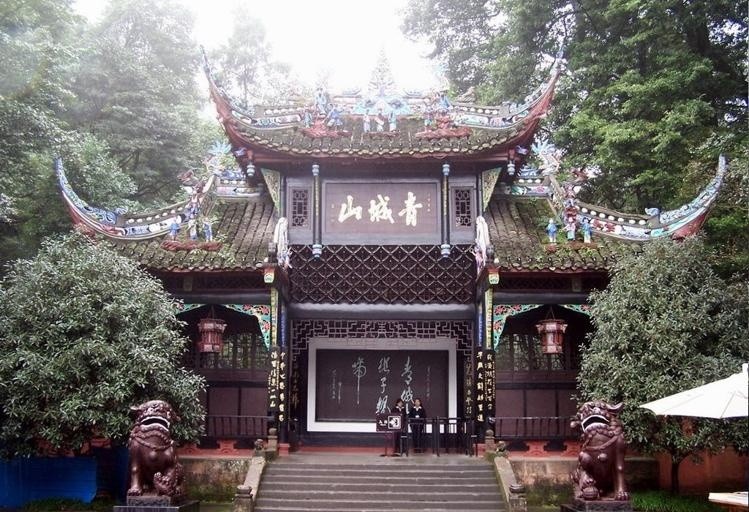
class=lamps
[197,305,228,354]
[535,306,568,355]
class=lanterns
[535,317,568,355]
[196,318,227,354]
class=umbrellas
[637,362,749,421]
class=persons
[545,183,593,245]
[303,85,454,132]
[169,193,220,242]
[410,398,426,448]
[391,398,409,452]
